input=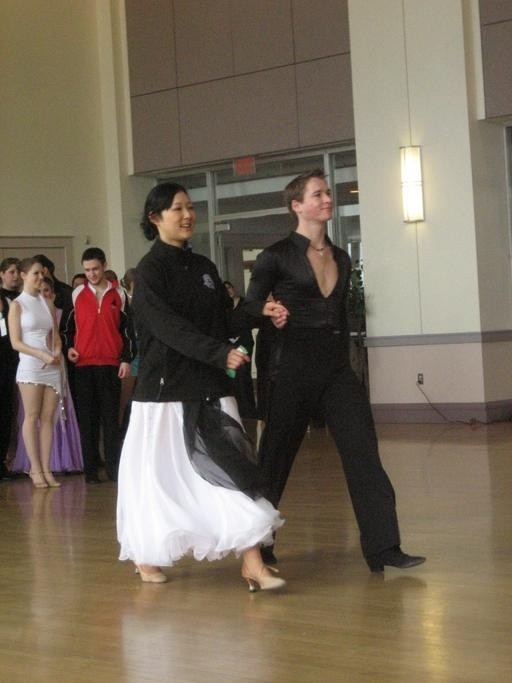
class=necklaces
[309,245,325,257]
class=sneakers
[0,463,11,480]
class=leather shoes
[259,544,278,565]
[365,546,427,573]
[84,475,103,484]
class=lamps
[398,145,425,223]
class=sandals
[29,469,62,489]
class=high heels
[131,557,168,582]
[241,562,288,593]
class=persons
[0,246,137,489]
[242,166,427,575]
[113,182,287,594]
[224,279,255,410]
